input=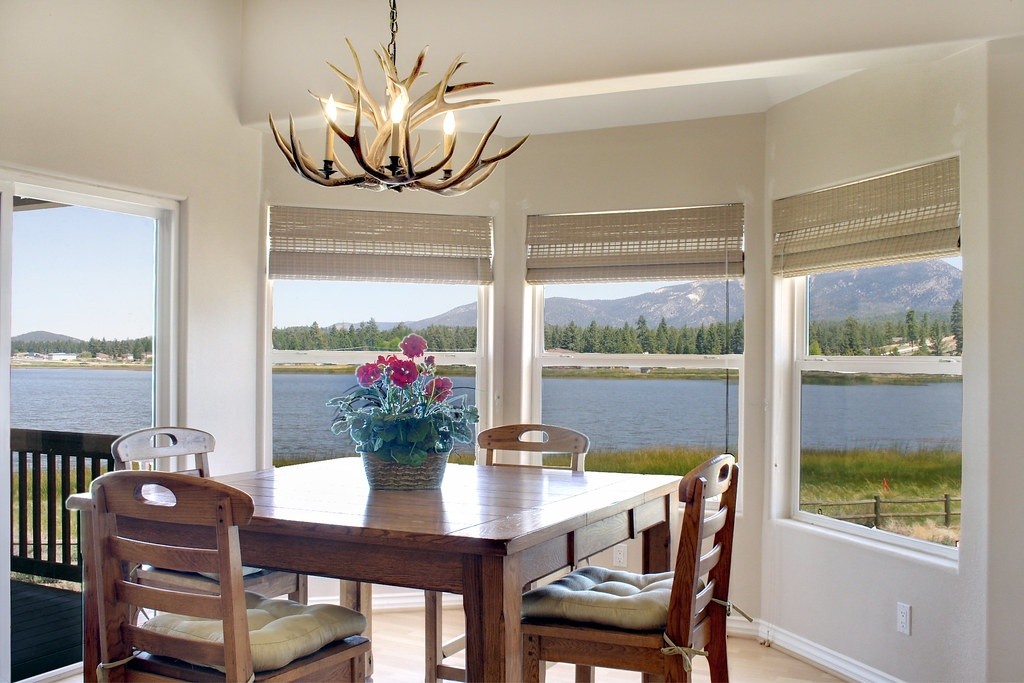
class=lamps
[268,0,530,198]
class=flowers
[324,332,480,469]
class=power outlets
[612,544,628,568]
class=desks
[69,456,685,683]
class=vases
[360,452,449,490]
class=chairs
[896,601,912,636]
[87,469,370,683]
[112,426,307,605]
[424,421,590,682]
[508,453,741,683]
